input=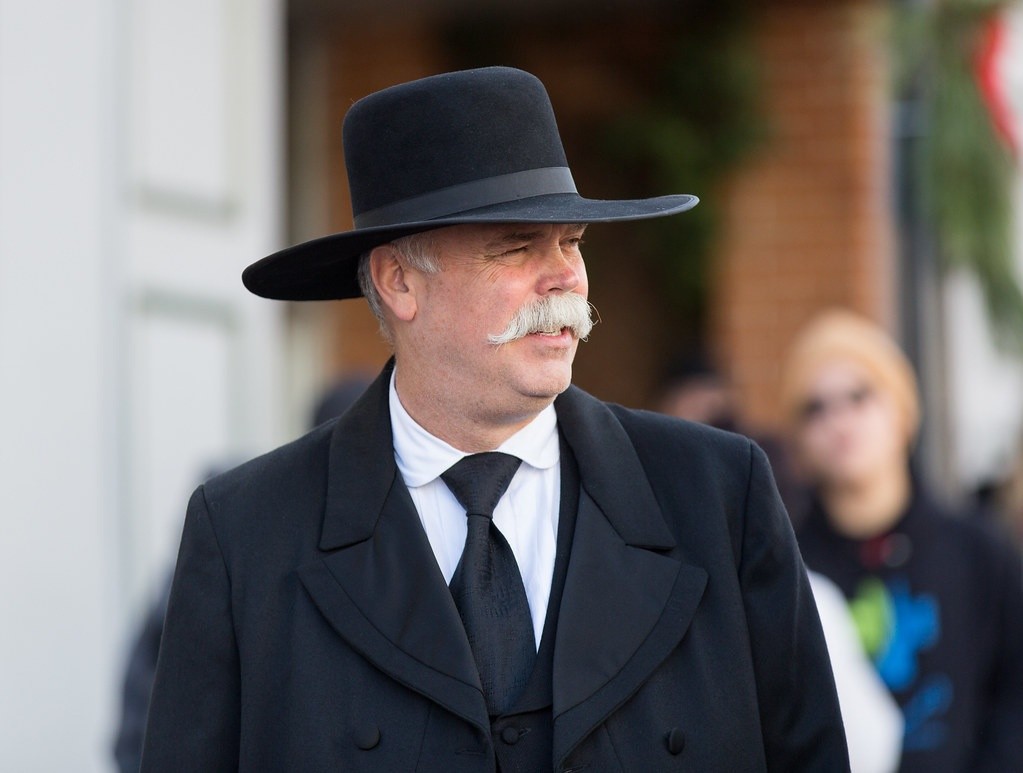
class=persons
[139,66,850,773]
[115,310,1023,773]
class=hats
[240,64,699,302]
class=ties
[439,451,537,719]
